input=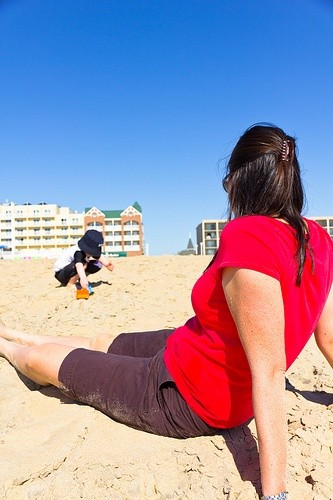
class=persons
[54,230,114,292]
[1,121,333,500]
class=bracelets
[261,492,287,500]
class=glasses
[222,172,231,193]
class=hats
[78,229,104,257]
